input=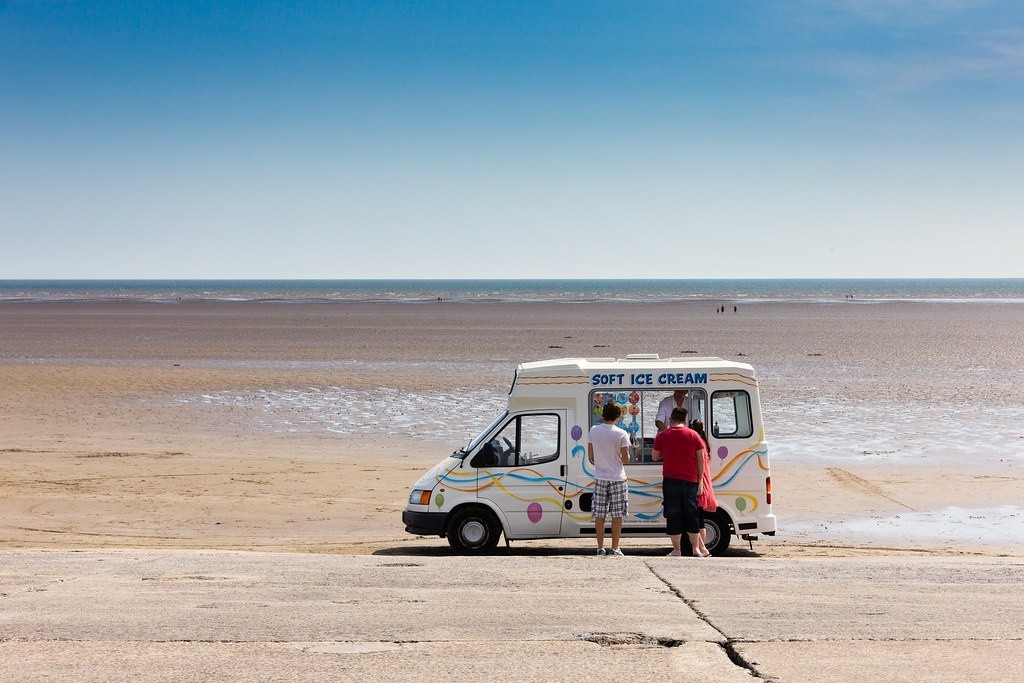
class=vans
[401,352,777,556]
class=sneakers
[597,549,606,556]
[609,548,624,556]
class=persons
[652,407,717,557]
[655,391,700,432]
[588,403,631,556]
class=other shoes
[667,553,680,556]
[693,553,711,557]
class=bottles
[714,421,720,437]
